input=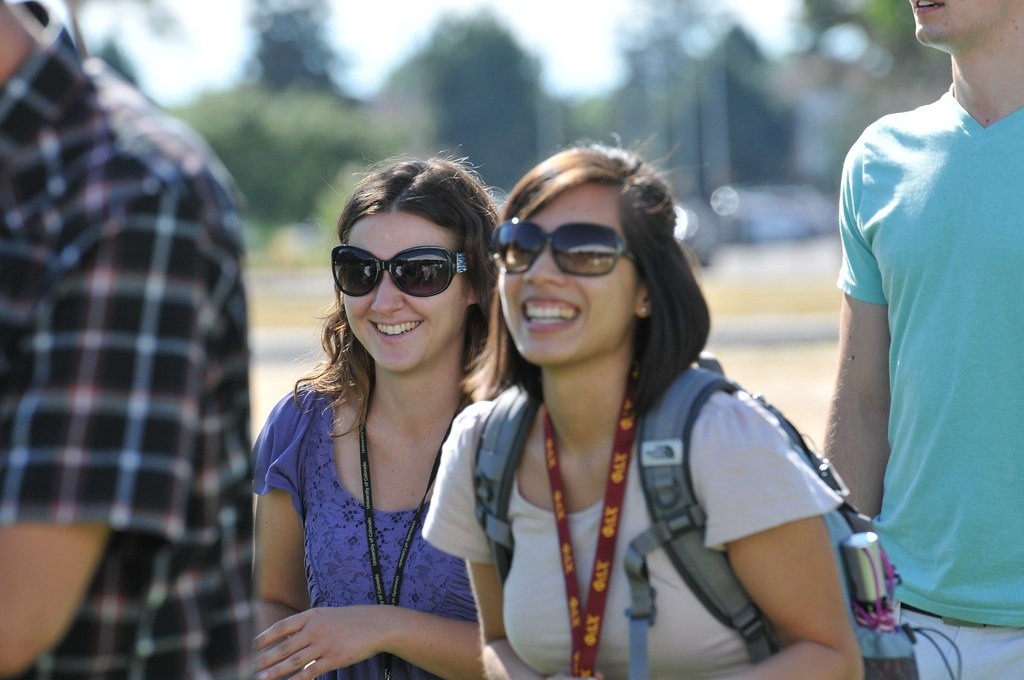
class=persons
[823,0,1024,680]
[421,142,918,680]
[248,157,510,678]
[0,1,260,680]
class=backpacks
[469,373,917,679]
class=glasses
[489,218,638,279]
[330,244,472,297]
